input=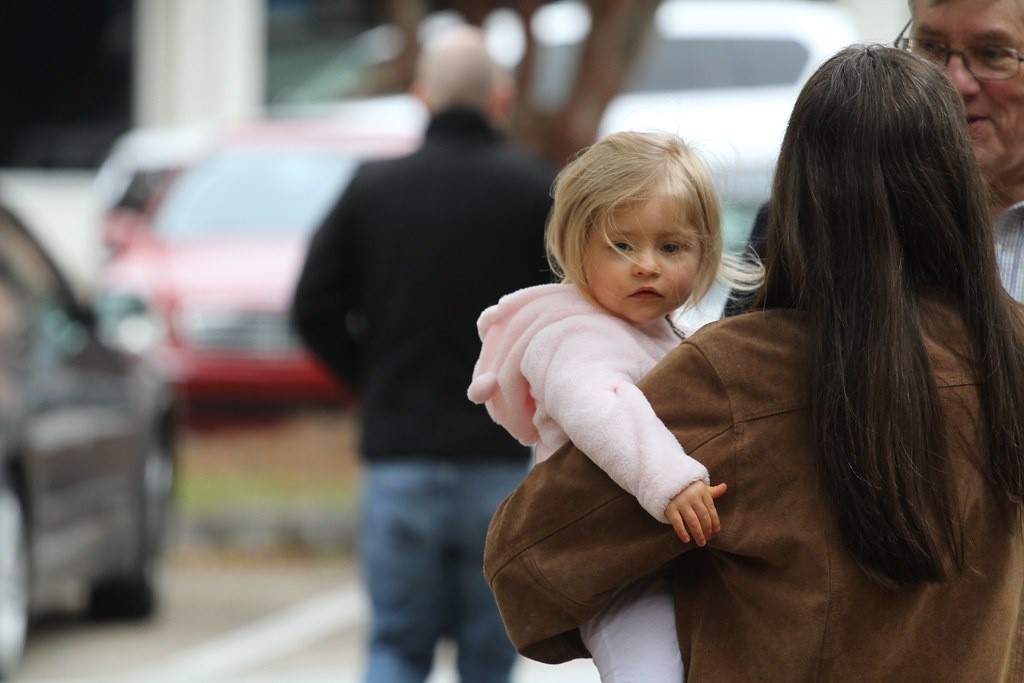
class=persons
[714,0,1024,337]
[288,20,559,683]
[483,42,1024,683]
[466,133,774,683]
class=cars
[0,203,191,683]
[84,127,424,415]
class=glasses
[894,17,1023,83]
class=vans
[288,4,858,279]
